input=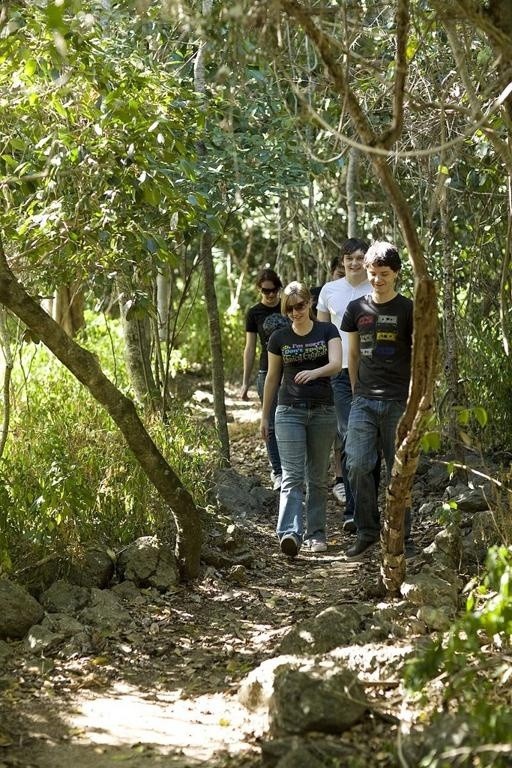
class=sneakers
[344,538,380,562]
[273,475,282,490]
[333,482,346,502]
[280,534,298,555]
[343,518,357,531]
[306,538,328,552]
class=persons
[259,282,342,555]
[242,268,293,489]
[339,242,414,557]
[316,237,374,532]
[309,257,349,503]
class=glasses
[286,299,305,312]
[261,287,278,294]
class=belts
[290,401,321,409]
